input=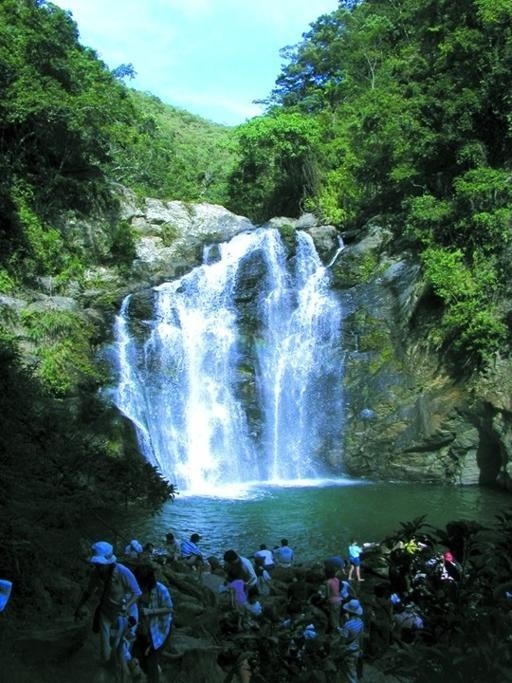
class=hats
[342,598,363,617]
[131,539,140,553]
[86,541,119,565]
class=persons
[201,550,271,617]
[115,593,143,680]
[255,539,294,570]
[126,531,202,565]
[349,541,365,581]
[337,598,364,681]
[281,605,316,638]
[389,537,457,642]
[76,541,142,661]
[325,556,348,580]
[131,565,173,681]
[326,575,356,606]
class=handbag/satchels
[356,657,366,679]
